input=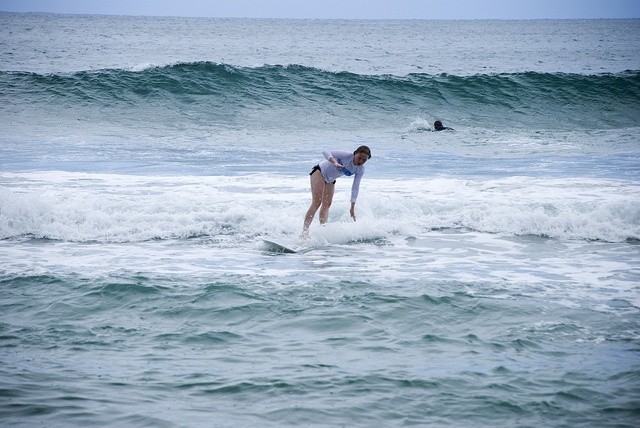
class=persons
[303,145,372,235]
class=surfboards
[261,239,298,254]
